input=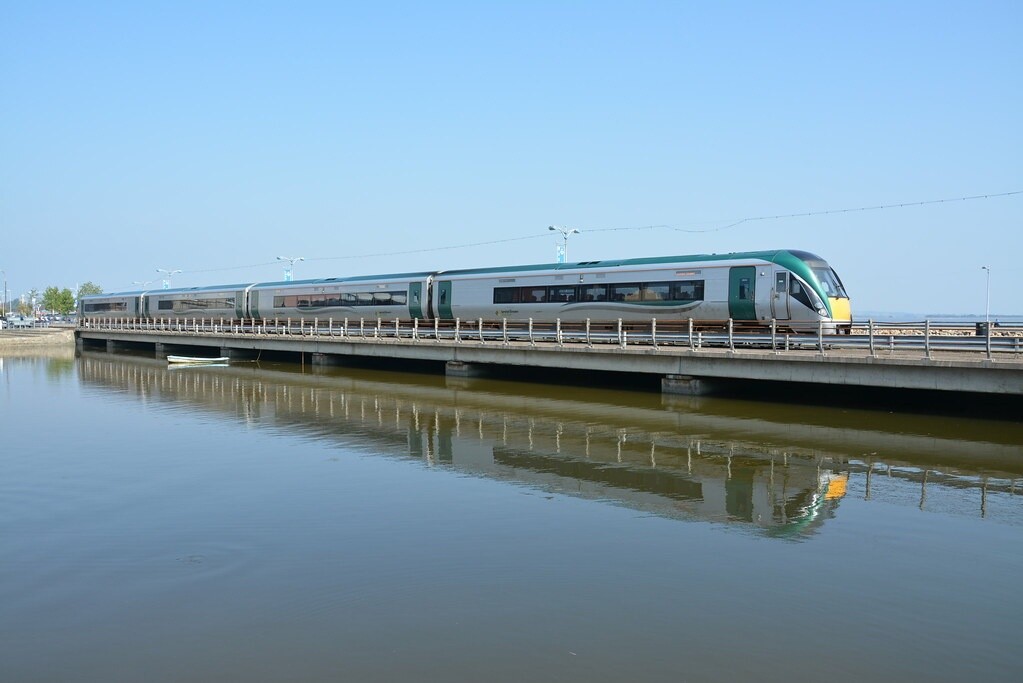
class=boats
[166,355,231,364]
[167,361,231,371]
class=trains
[70,348,852,532]
[76,247,854,342]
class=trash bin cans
[976,322,988,335]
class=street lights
[155,269,182,289]
[276,255,304,282]
[981,266,990,321]
[548,226,580,262]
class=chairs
[532,291,690,302]
